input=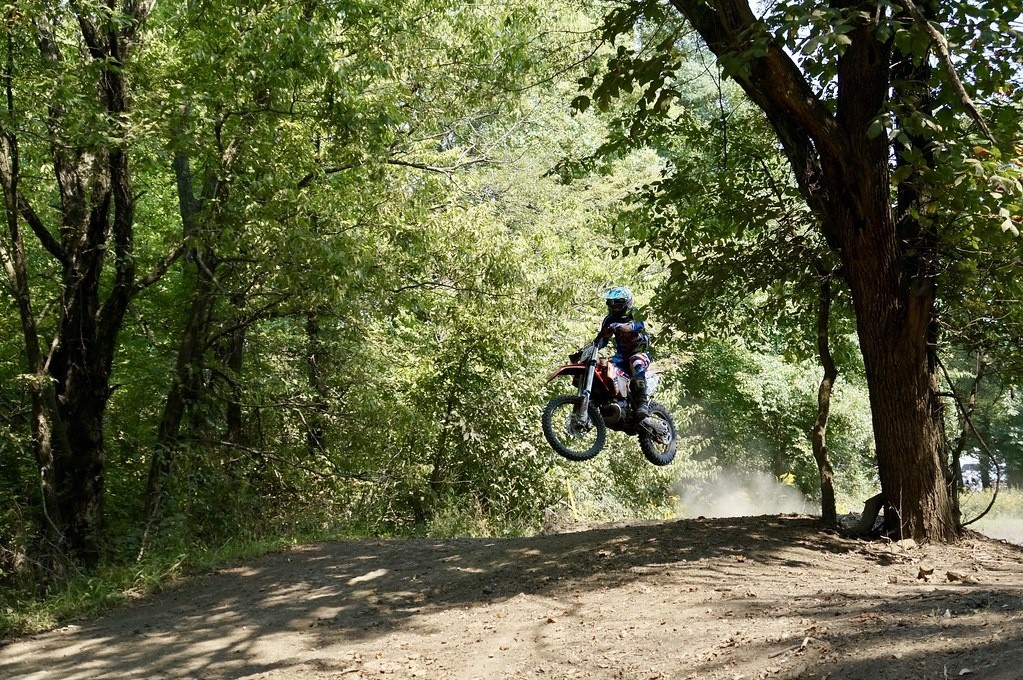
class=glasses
[606,298,626,306]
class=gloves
[607,323,633,337]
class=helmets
[605,287,633,312]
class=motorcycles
[541,325,678,466]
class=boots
[634,377,648,414]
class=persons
[586,287,656,416]
